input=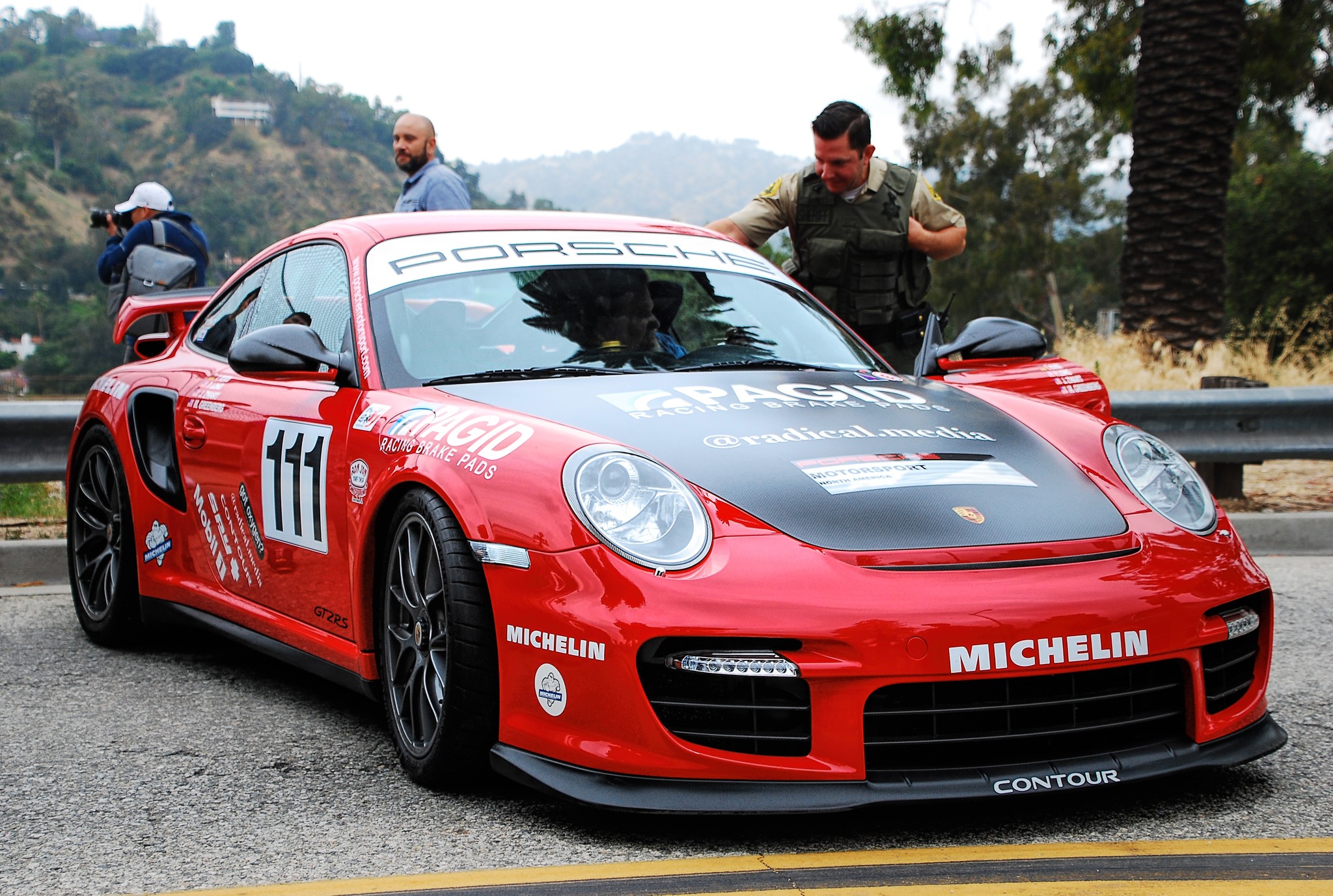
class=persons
[202,286,312,358]
[392,114,472,213]
[703,100,967,375]
[543,266,690,368]
[95,182,210,363]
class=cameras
[89,209,134,231]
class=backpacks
[106,218,198,339]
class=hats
[115,180,175,215]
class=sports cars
[63,209,1291,817]
